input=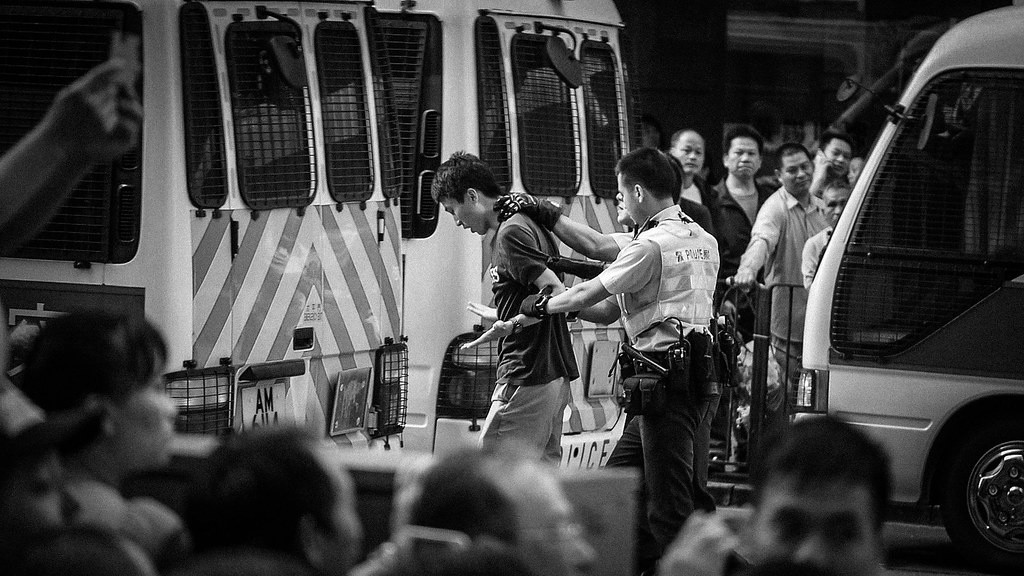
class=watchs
[509,317,521,334]
[535,294,553,319]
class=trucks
[793,0,1024,576]
[1,1,410,455]
[373,1,634,473]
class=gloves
[494,192,561,232]
[519,286,553,318]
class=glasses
[516,518,583,547]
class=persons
[430,152,580,466]
[519,148,720,576]
[733,145,829,378]
[0,307,893,576]
[710,124,774,345]
[809,131,850,197]
[669,128,719,224]
[847,155,863,185]
[802,181,850,288]
[493,161,717,511]
